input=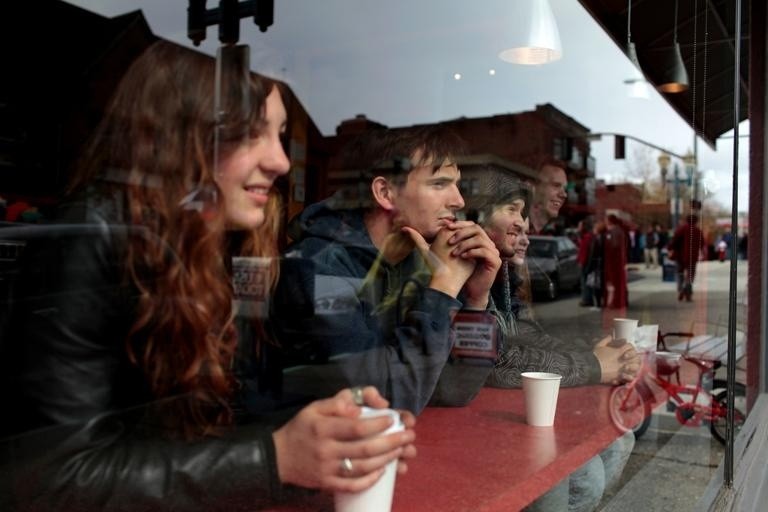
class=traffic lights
[614,131,624,161]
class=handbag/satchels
[585,270,601,288]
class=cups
[528,423,558,478]
[518,369,561,428]
[331,403,403,512]
[612,315,637,344]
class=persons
[2,30,419,512]
[458,170,639,389]
[575,212,749,330]
[509,213,531,305]
[527,159,595,348]
[258,116,502,415]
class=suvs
[2,222,178,348]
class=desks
[197,334,713,509]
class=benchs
[660,311,748,408]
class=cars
[508,230,582,306]
[297,239,384,340]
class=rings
[342,456,355,477]
[349,384,363,406]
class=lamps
[658,0,691,94]
[499,0,564,67]
[615,0,649,86]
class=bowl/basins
[654,350,681,366]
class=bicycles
[605,324,746,450]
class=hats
[465,171,533,226]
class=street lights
[654,144,695,234]
[619,71,701,207]
